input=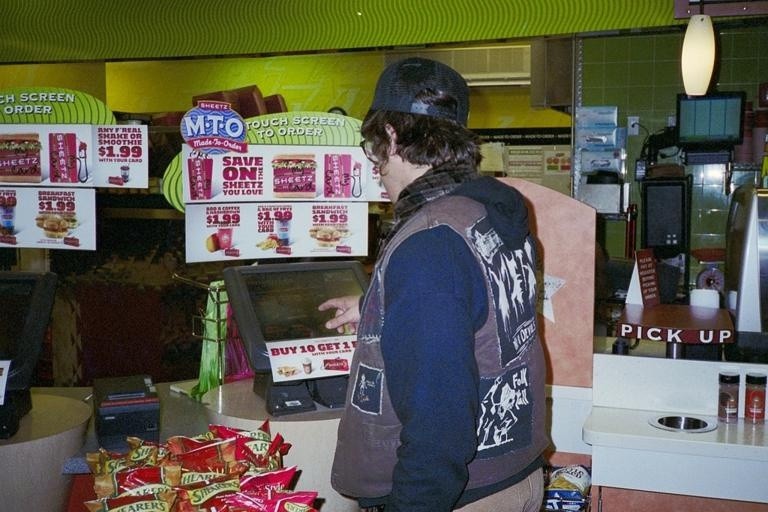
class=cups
[733,100,768,164]
[302,361,312,373]
[218,227,232,249]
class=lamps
[681,0,717,99]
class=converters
[635,160,646,181]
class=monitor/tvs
[677,91,746,148]
[224,260,370,415]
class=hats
[361,58,469,138]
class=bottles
[108,176,124,186]
[120,166,129,182]
[64,236,79,247]
[275,246,292,255]
[225,249,240,258]
[278,219,289,245]
[336,246,352,253]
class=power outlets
[627,116,640,137]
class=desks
[1,393,92,512]
[202,378,359,512]
[616,303,736,358]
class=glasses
[360,139,380,164]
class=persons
[318,56,554,512]
